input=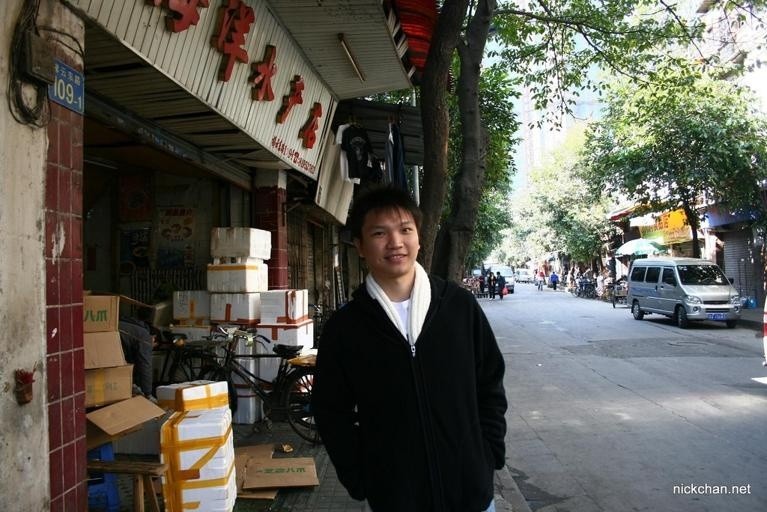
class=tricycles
[570,274,627,309]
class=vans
[627,257,743,329]
[486,264,515,294]
[515,269,529,283]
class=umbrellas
[614,238,666,258]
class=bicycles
[143,320,326,447]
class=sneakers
[489,298,495,300]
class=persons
[312,187,507,512]
[468,272,506,302]
[538,272,544,291]
[119,317,161,401]
[550,272,558,290]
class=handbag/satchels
[502,288,509,295]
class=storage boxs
[74,223,321,511]
